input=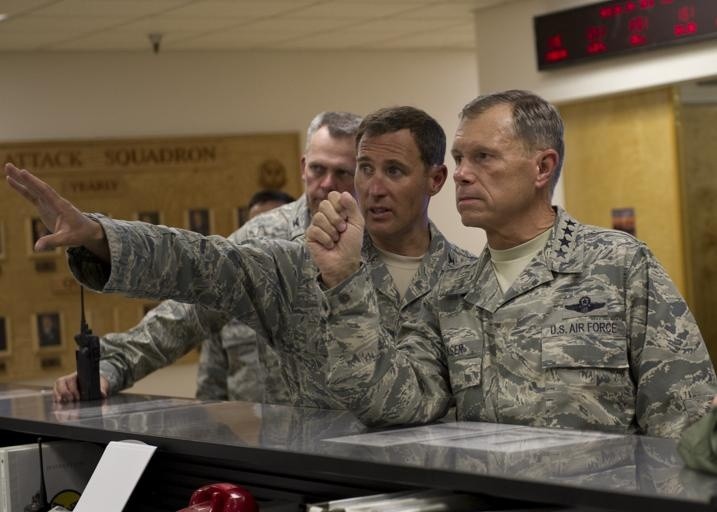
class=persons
[197,190,294,404]
[306,90,717,440]
[5,105,477,411]
[50,110,364,405]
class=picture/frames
[0,205,250,358]
